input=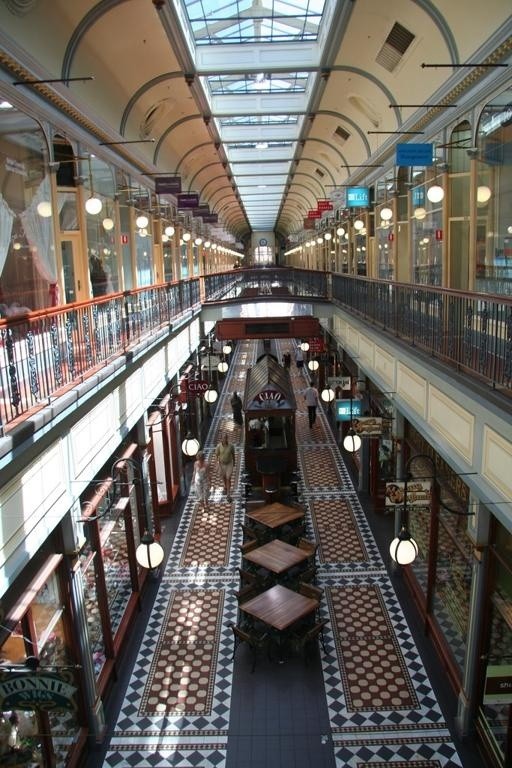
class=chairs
[227,492,330,675]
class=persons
[194,452,211,513]
[231,391,243,425]
[304,382,318,429]
[216,432,235,502]
[294,347,305,373]
[283,350,291,369]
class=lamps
[77,336,231,570]
[283,137,481,256]
[307,359,320,372]
[53,157,245,261]
[300,342,309,352]
[381,471,477,565]
[321,356,360,402]
[343,390,397,452]
